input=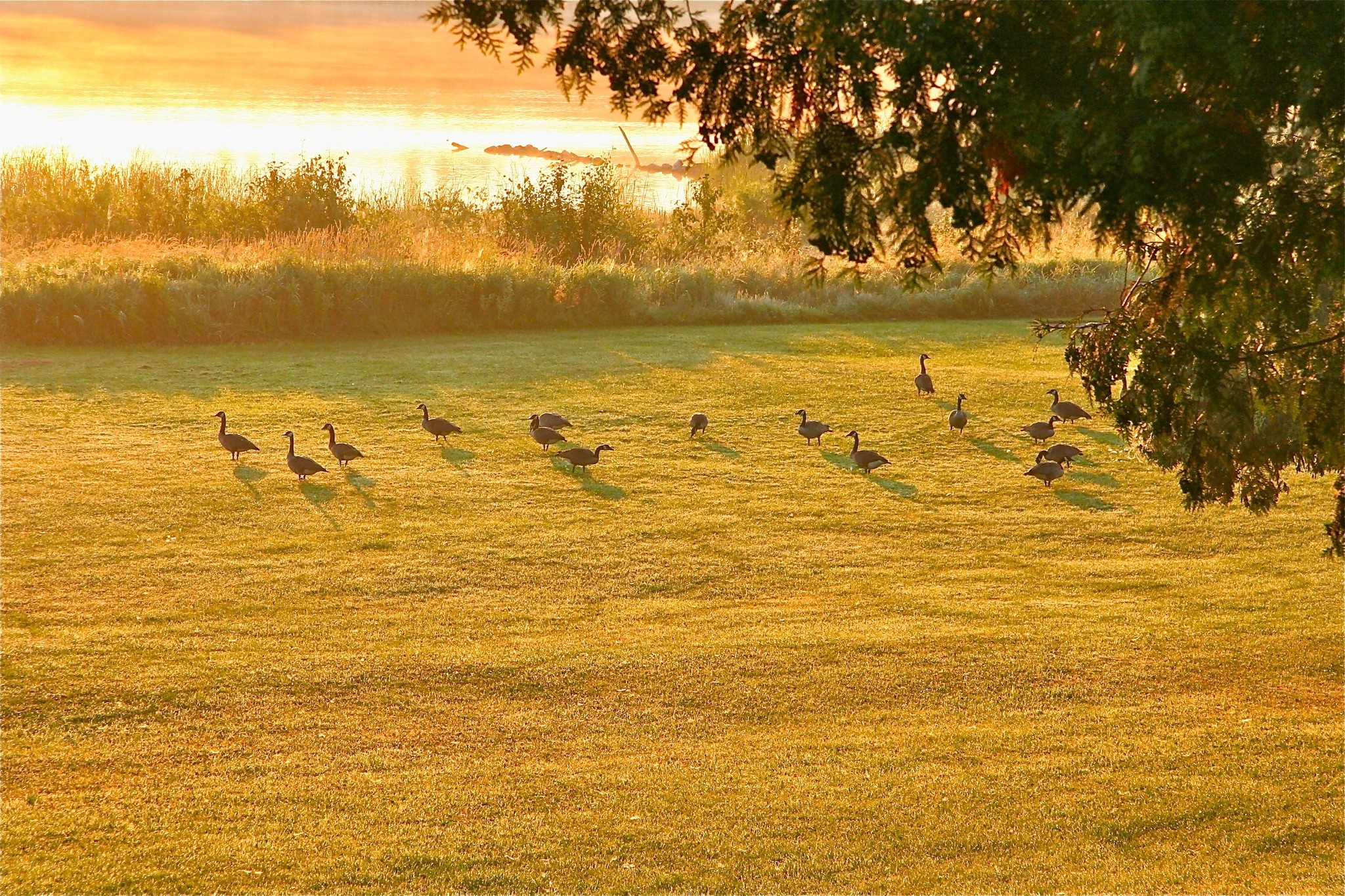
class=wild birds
[689,413,708,438]
[947,394,967,434]
[416,404,462,441]
[846,430,892,475]
[322,424,365,467]
[795,409,833,446]
[554,444,614,473]
[1120,377,1128,397]
[1035,444,1083,468]
[528,414,567,451]
[213,411,260,460]
[530,413,573,434]
[1046,389,1092,424]
[282,431,329,480]
[1021,416,1062,445]
[1024,456,1075,487]
[914,353,935,395]
